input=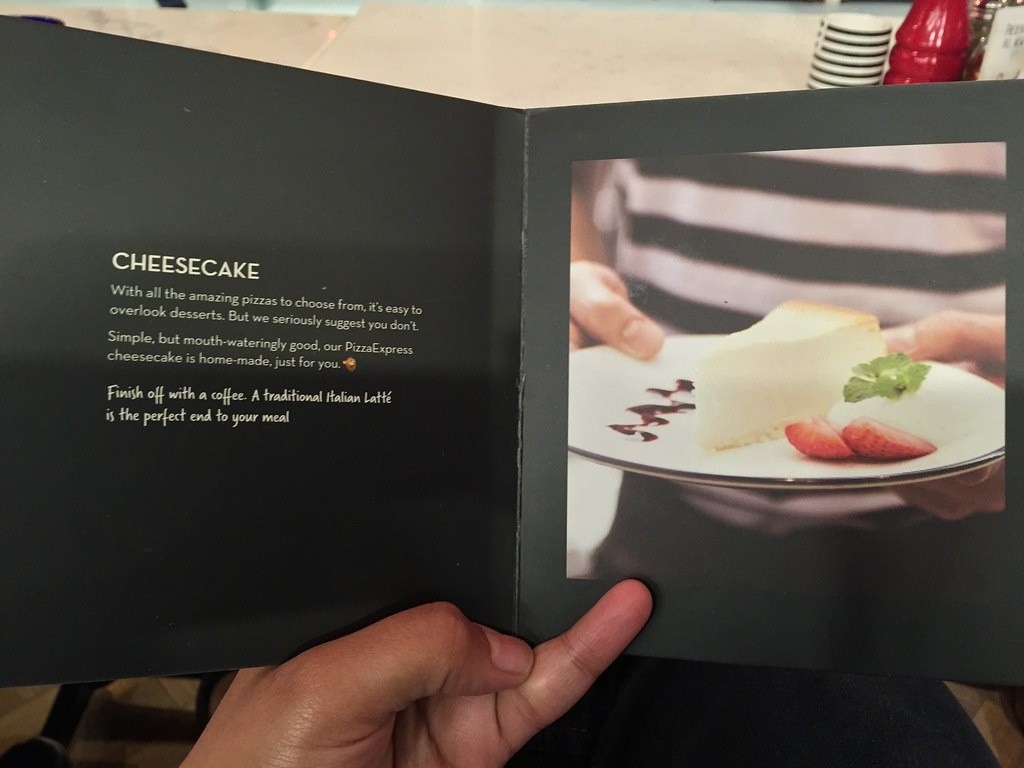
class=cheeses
[695,301,885,447]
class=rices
[827,359,1005,446]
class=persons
[568,143,1006,615]
[179,578,654,768]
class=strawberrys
[785,416,937,459]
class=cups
[806,12,893,91]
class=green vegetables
[844,352,931,402]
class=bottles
[962,0,1005,82]
[883,1,970,85]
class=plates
[566,334,1007,489]
